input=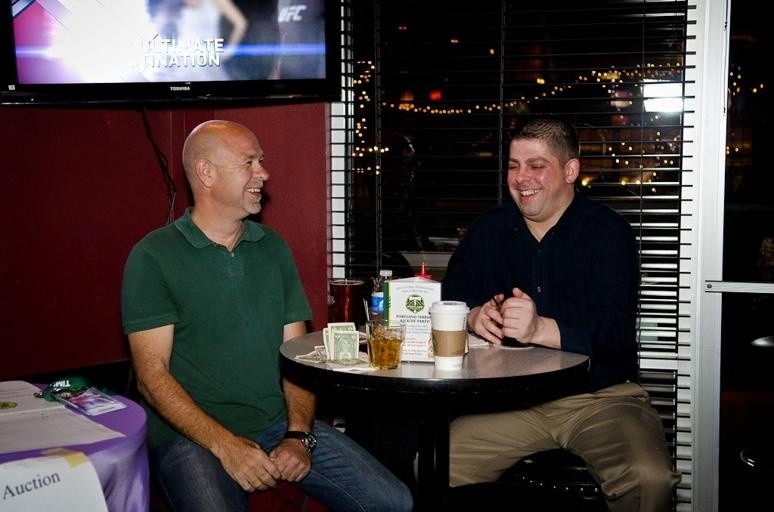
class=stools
[518,448,598,510]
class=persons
[121,120,414,512]
[143,2,248,81]
[438,115,680,512]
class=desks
[0,381,148,512]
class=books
[58,387,128,416]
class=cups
[428,300,471,371]
[365,321,407,371]
[329,278,364,327]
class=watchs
[282,430,319,452]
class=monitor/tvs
[0,0,342,112]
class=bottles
[371,270,392,325]
